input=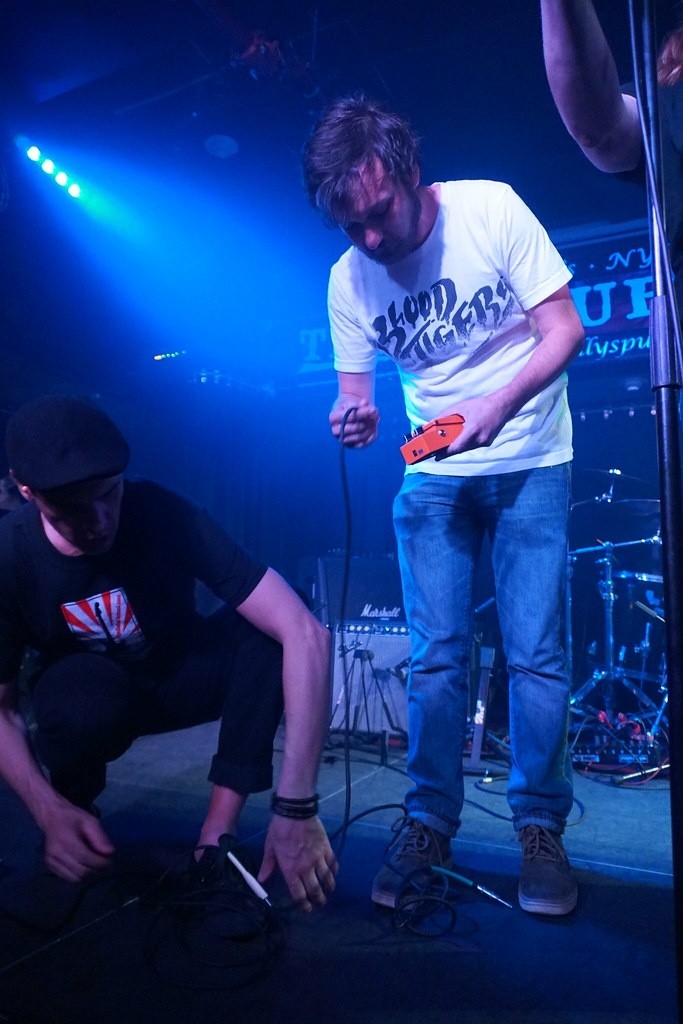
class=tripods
[563,530,671,756]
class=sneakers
[518,823,578,915]
[371,815,452,910]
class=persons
[301,95,585,915]
[0,395,339,914]
[540,0,683,344]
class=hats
[6,396,130,491]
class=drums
[586,569,665,683]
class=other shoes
[188,834,266,938]
[15,803,100,931]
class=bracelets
[271,791,319,819]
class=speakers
[307,549,481,742]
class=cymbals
[570,498,661,526]
[576,466,658,493]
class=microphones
[385,667,407,681]
